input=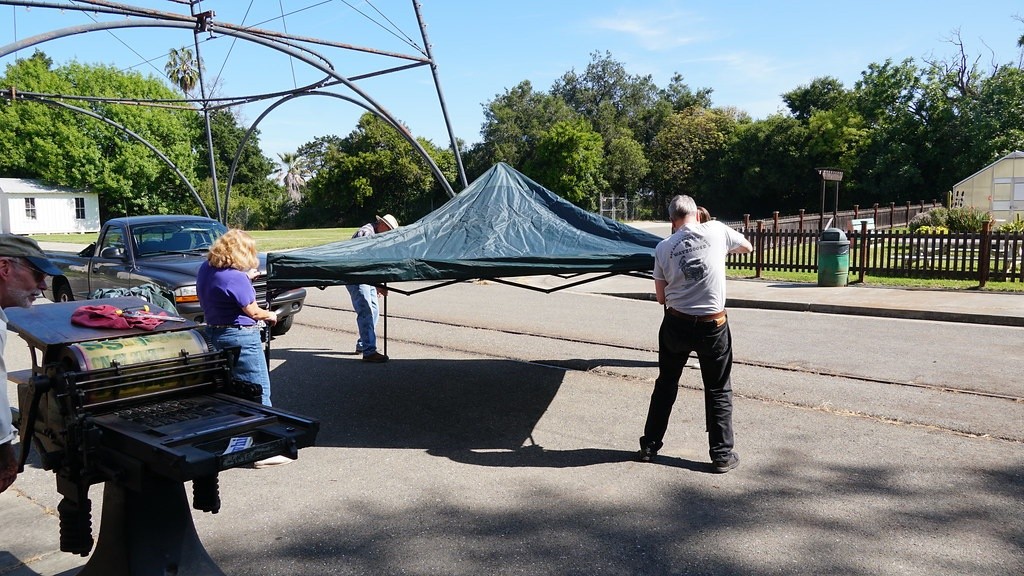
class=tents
[266,162,665,456]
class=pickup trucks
[38,214,306,335]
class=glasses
[8,258,46,282]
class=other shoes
[638,449,656,461]
[713,452,740,472]
[363,352,389,363]
[255,455,295,469]
[354,348,363,355]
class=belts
[208,325,252,329]
[668,307,726,321]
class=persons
[0,234,63,494]
[692,206,711,369]
[637,195,753,473]
[344,214,398,363]
[196,230,292,469]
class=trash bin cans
[816,228,851,286]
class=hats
[376,214,399,230]
[0,233,64,276]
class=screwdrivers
[112,305,150,316]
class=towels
[71,304,166,331]
